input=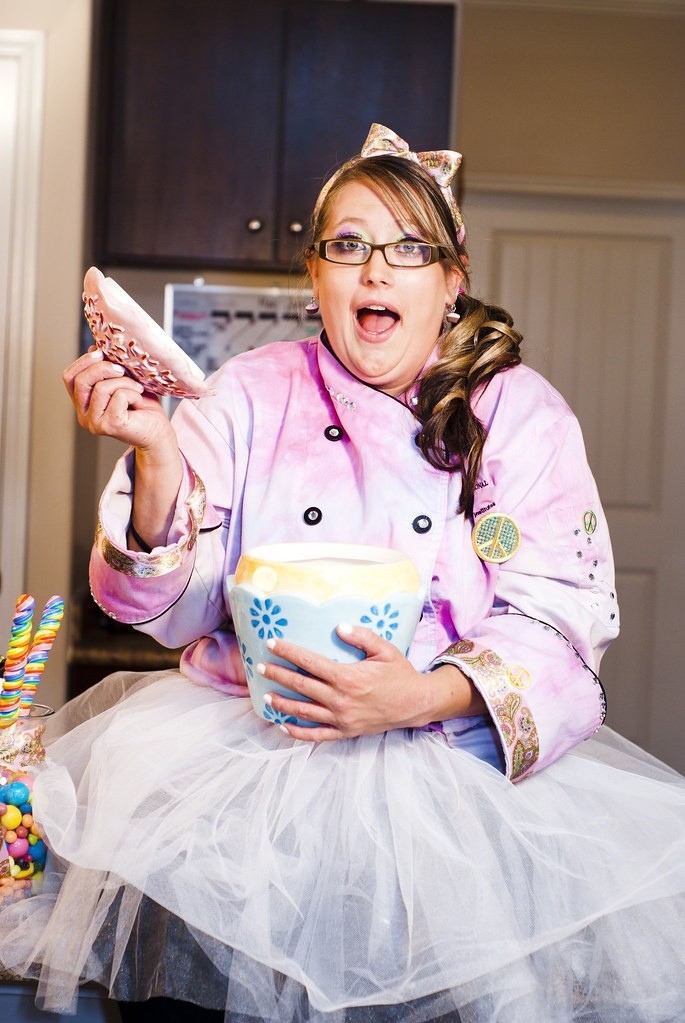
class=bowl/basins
[225,575,425,732]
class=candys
[0,595,65,911]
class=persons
[1,121,684,1023]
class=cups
[0,703,54,911]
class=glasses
[311,239,446,268]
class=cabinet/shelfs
[100,0,454,274]
